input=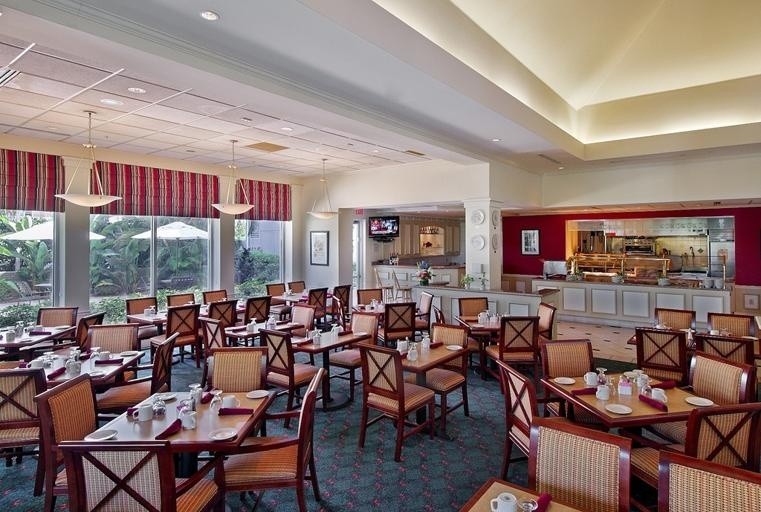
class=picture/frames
[521,229,540,255]
[309,230,329,266]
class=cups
[146,290,388,344]
[29,351,111,376]
[131,395,241,429]
[478,309,509,326]
[5,321,41,339]
[490,491,516,512]
[584,368,668,404]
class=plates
[208,430,237,440]
[685,396,715,407]
[118,350,138,356]
[446,345,465,351]
[554,377,576,385]
[84,429,118,443]
[606,403,632,415]
[247,390,270,399]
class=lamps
[210,139,255,215]
[306,157,341,219]
[54,108,123,208]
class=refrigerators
[708,240,736,279]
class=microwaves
[622,238,656,256]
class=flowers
[411,260,437,282]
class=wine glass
[88,347,100,370]
[517,495,538,512]
[209,389,223,411]
[188,383,202,407]
[421,333,431,358]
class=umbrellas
[130,221,208,275]
[0,221,106,240]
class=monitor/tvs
[368,215,399,243]
